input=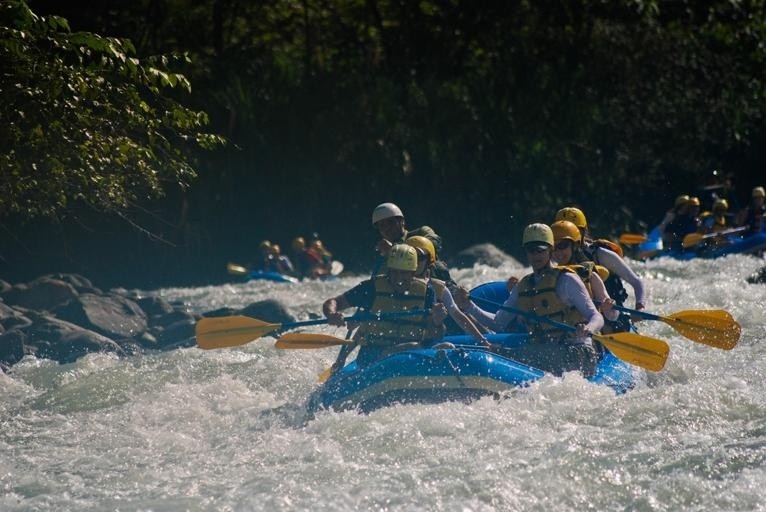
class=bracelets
[636,303,645,309]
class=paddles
[195,310,430,350]
[618,311,732,320]
[275,334,355,349]
[318,254,384,384]
[467,293,668,372]
[620,234,658,244]
[683,226,750,247]
[591,300,741,350]
[229,263,249,275]
[638,251,667,260]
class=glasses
[551,242,571,251]
[527,245,548,252]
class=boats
[639,207,766,264]
[241,268,340,285]
[304,280,638,419]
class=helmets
[269,245,280,256]
[673,194,689,209]
[712,199,729,211]
[554,208,588,229]
[372,203,404,225]
[404,236,437,264]
[685,197,700,208]
[385,244,418,272]
[523,223,554,248]
[312,241,323,250]
[551,221,581,248]
[260,240,272,250]
[293,238,306,248]
[752,187,766,197]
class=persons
[406,233,503,355]
[369,203,456,286]
[662,195,689,243]
[555,206,648,334]
[406,225,496,336]
[505,221,619,357]
[740,184,765,227]
[678,198,704,247]
[322,243,458,369]
[458,222,603,380]
[703,199,731,234]
[248,234,333,278]
